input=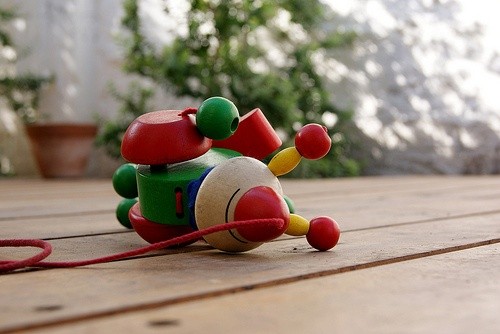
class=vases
[26,120,97,179]
